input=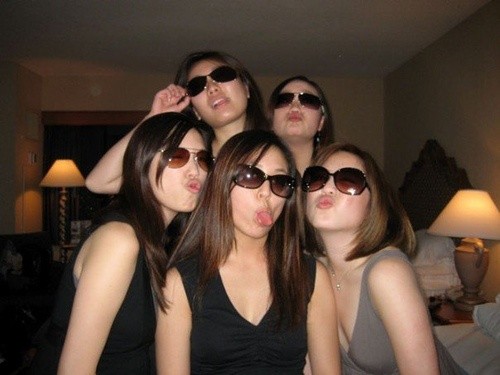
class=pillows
[413,228,457,267]
[472,303,500,343]
[0,239,23,280]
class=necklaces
[328,259,358,289]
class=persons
[86,48,335,195]
[26,111,218,375]
[301,142,471,375]
[155,129,343,375]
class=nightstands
[432,303,473,324]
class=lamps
[424,188,500,313]
[39,158,86,264]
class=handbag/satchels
[399,139,473,247]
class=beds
[434,323,500,375]
[397,138,473,306]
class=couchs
[0,231,54,351]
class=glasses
[186,66,237,96]
[301,165,371,195]
[156,147,216,172]
[273,92,320,110]
[232,164,298,199]
